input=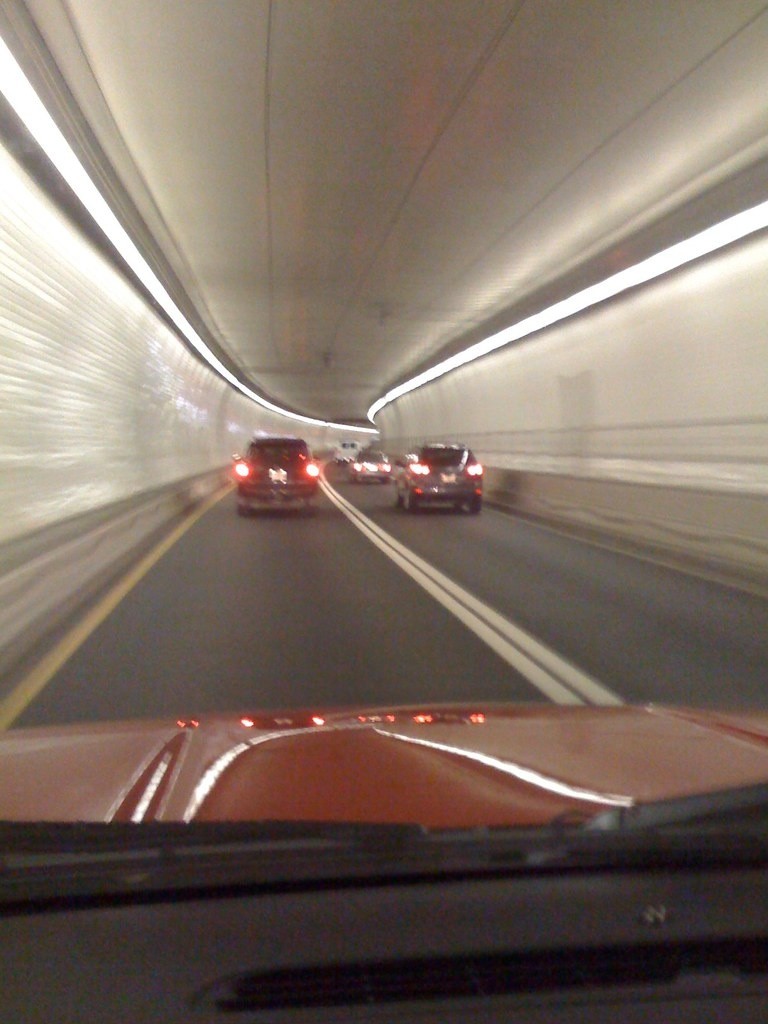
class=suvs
[235,438,320,519]
[394,441,482,515]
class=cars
[349,448,391,485]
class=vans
[336,440,361,465]
